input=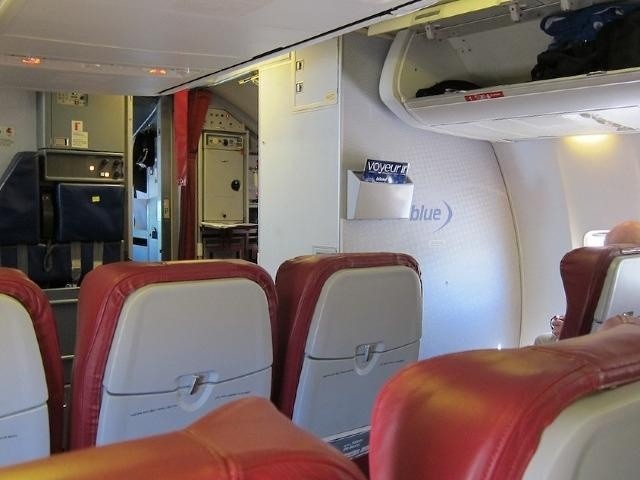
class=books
[360,158,409,184]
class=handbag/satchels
[414,79,482,98]
[526,0,640,82]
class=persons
[533,218,640,346]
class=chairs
[275,250,423,460]
[2,399,362,478]
[557,245,637,337]
[3,269,68,468]
[69,259,284,449]
[363,319,638,477]
